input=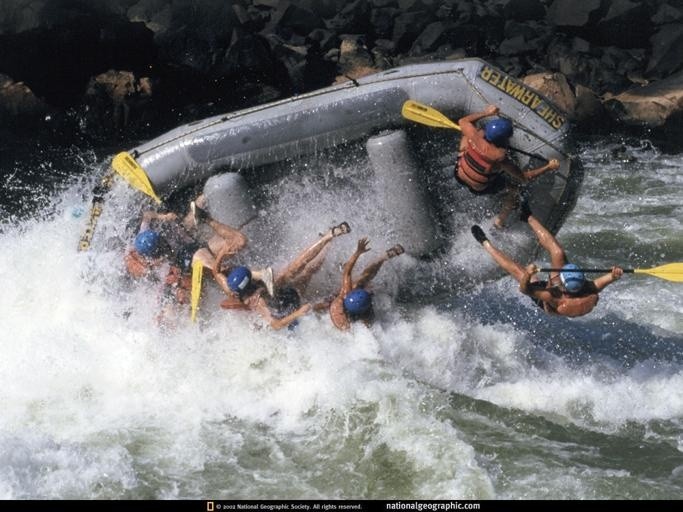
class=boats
[79,57,585,308]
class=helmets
[559,264,585,294]
[227,266,251,293]
[485,117,513,143]
[135,229,160,255]
[345,287,371,316]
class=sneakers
[520,198,532,221]
[262,267,275,297]
[190,201,205,225]
[471,225,489,244]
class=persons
[124,196,405,331]
[455,105,559,235]
[470,198,624,318]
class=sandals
[387,244,405,258]
[330,221,351,236]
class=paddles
[189,259,203,322]
[112,152,204,247]
[534,263,683,285]
[402,100,551,167]
